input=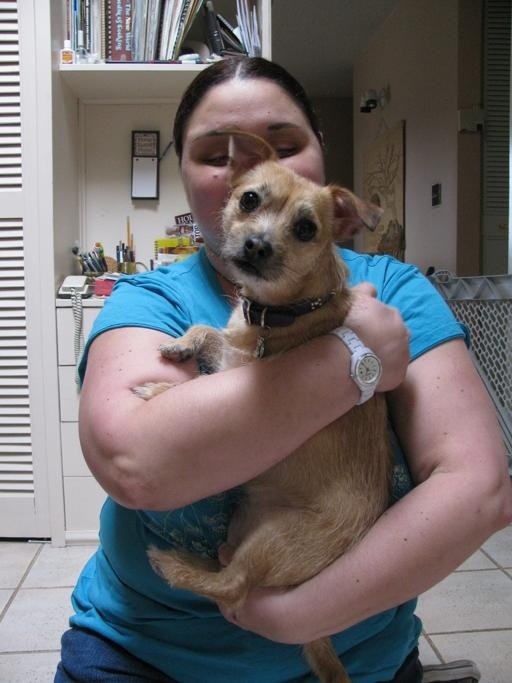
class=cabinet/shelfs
[51,0,273,548]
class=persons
[50,59,512,683]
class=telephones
[58,275,94,298]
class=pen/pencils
[72,242,108,272]
[117,216,135,263]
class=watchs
[330,323,382,408]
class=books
[50,0,262,64]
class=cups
[118,262,136,275]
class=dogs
[127,126,391,683]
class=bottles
[59,40,76,65]
